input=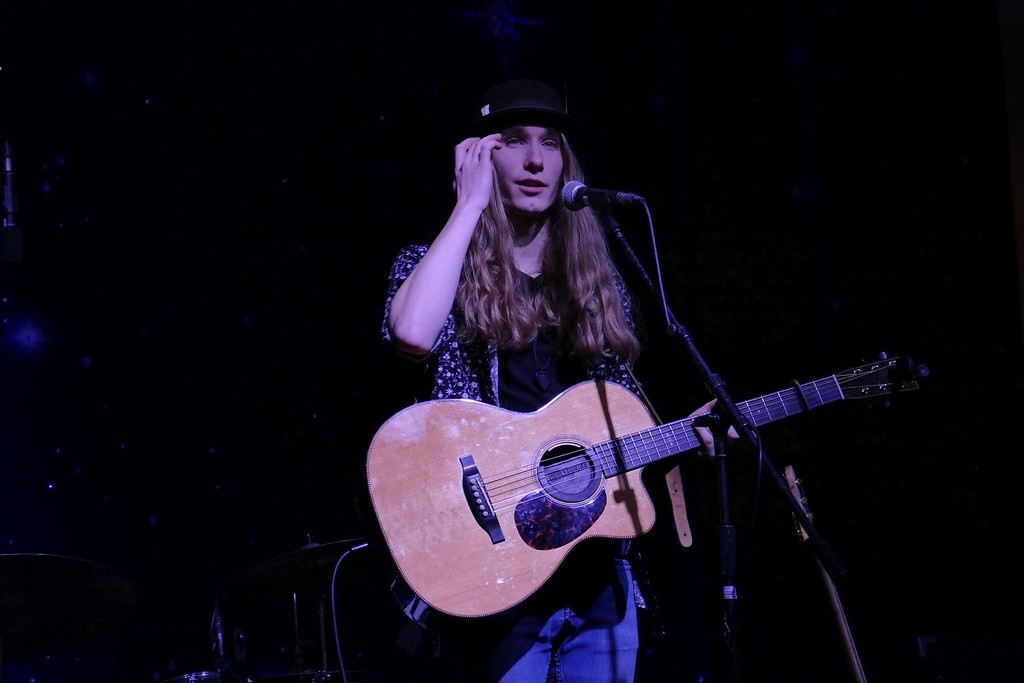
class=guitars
[365,350,927,629]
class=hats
[463,78,570,133]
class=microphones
[561,180,643,211]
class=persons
[376,75,747,681]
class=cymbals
[257,538,364,571]
